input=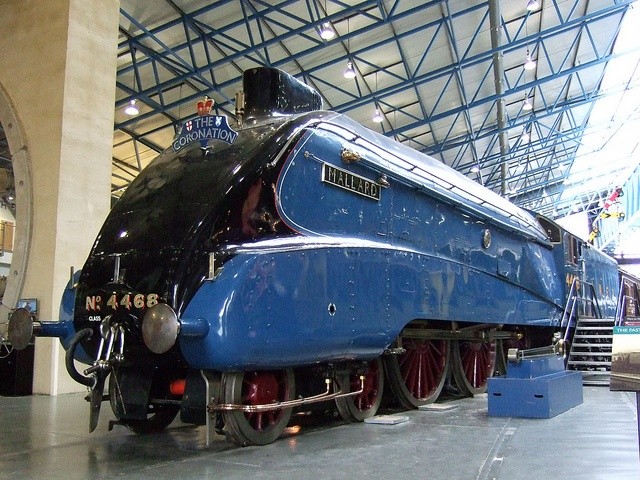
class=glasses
[522,94,531,111]
[470,165,478,173]
[320,0,334,39]
[527,1,539,10]
[524,50,535,70]
[373,70,383,123]
[123,71,141,117]
[344,18,356,79]
